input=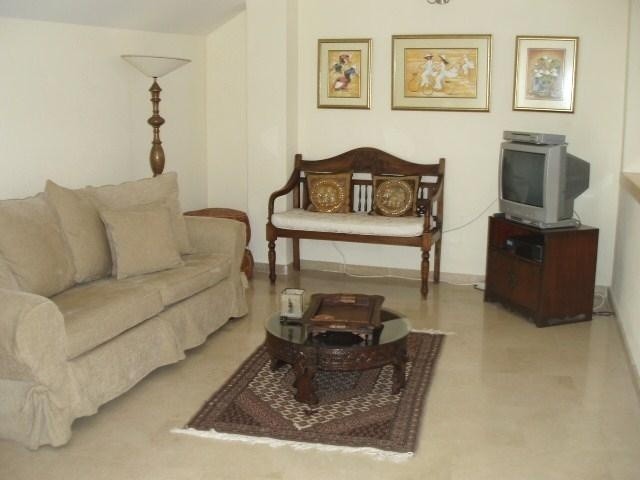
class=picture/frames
[317,38,371,108]
[512,35,578,113]
[390,34,492,112]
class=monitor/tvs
[498,140,590,230]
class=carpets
[169,328,457,464]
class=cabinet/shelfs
[484,215,599,327]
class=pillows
[367,174,421,218]
[302,169,355,213]
[1,191,79,296]
[98,208,188,280]
[86,171,198,275]
[46,180,113,283]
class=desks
[183,207,253,282]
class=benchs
[265,147,445,300]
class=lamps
[121,55,191,177]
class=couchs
[0,216,249,451]
